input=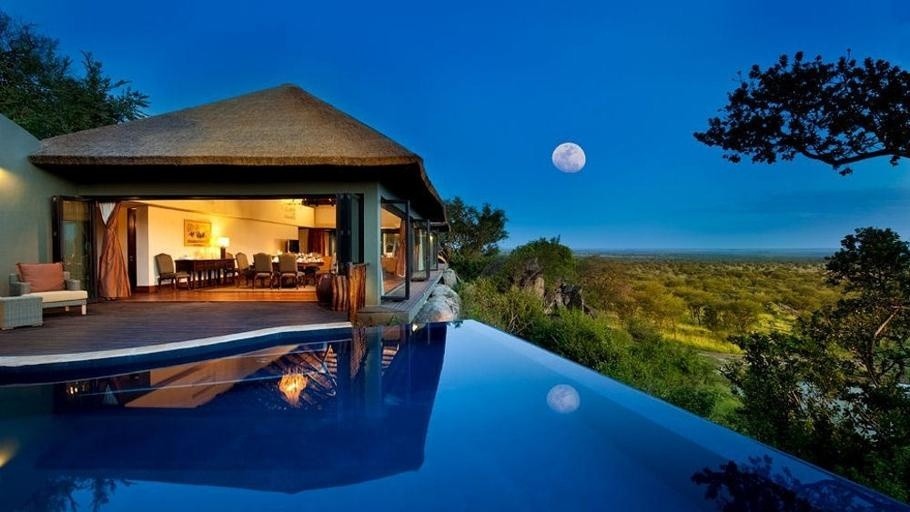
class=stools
[1,295,44,331]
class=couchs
[8,262,88,315]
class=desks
[175,259,234,290]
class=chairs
[235,251,336,290]
[223,253,239,282]
[155,254,191,292]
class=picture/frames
[184,219,213,248]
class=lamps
[218,236,229,259]
[279,373,307,406]
